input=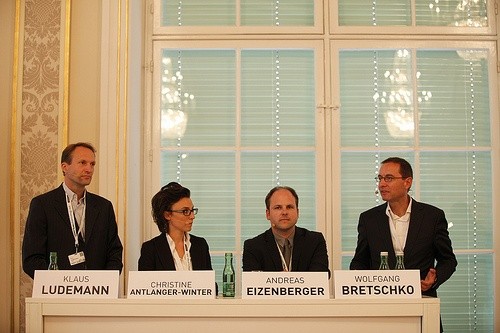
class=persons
[240,185,331,280]
[138,182,219,295]
[350,157,458,333]
[22,141,124,279]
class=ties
[282,239,291,271]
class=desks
[25,296,440,333]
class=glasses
[375,176,406,182]
[170,208,198,215]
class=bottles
[47,252,59,270]
[394,250,406,270]
[379,251,390,269]
[222,254,235,298]
